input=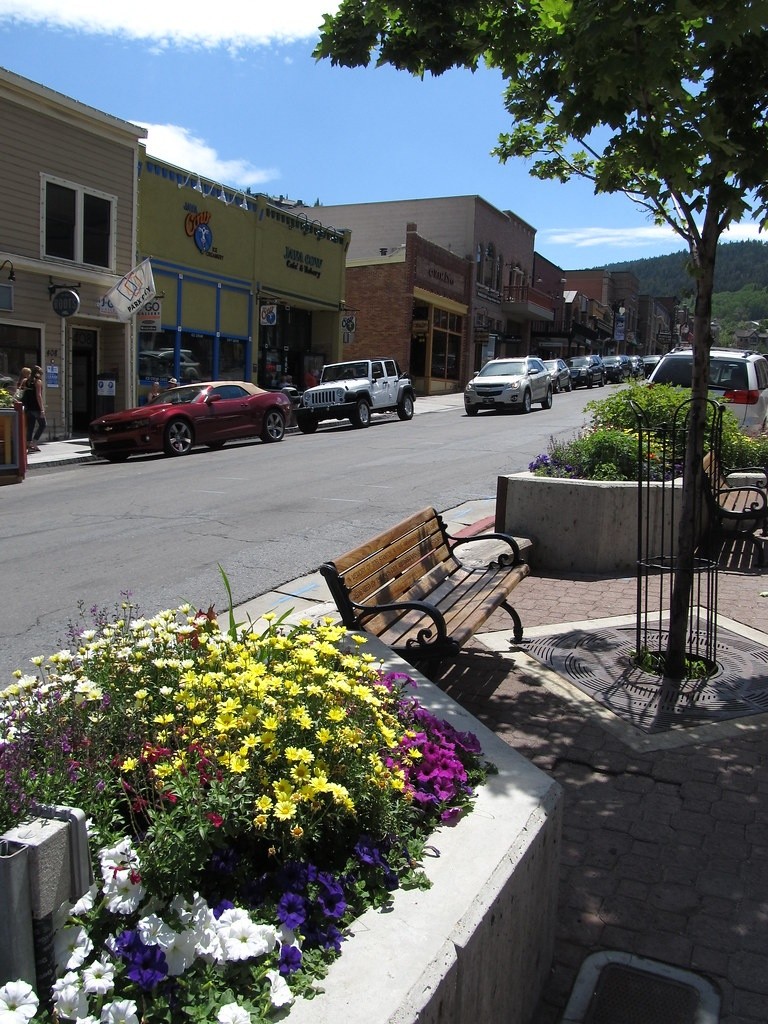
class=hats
[168,378,177,385]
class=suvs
[295,358,417,434]
[564,354,606,389]
[464,355,553,415]
[642,355,663,375]
[601,356,633,383]
[644,348,768,437]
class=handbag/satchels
[14,378,27,400]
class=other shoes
[28,445,41,453]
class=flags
[106,257,157,321]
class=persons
[1,365,47,451]
[304,366,317,391]
[147,377,179,404]
[281,374,295,388]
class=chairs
[318,505,532,685]
[698,448,768,540]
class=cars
[89,380,291,462]
[138,350,200,382]
[542,359,572,394]
